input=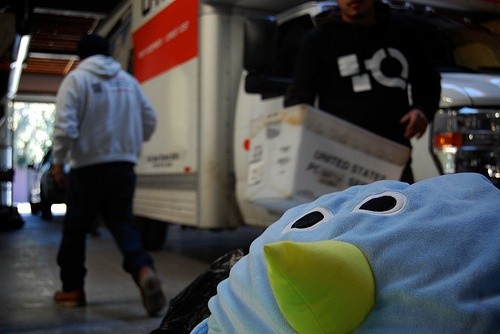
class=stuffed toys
[189,171,500,334]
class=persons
[50,32,169,316]
[284,1,443,183]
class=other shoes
[53,287,89,309]
[139,266,167,316]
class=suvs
[27,149,67,222]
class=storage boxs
[244,103,411,213]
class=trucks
[88,0,500,256]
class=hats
[73,34,110,57]
